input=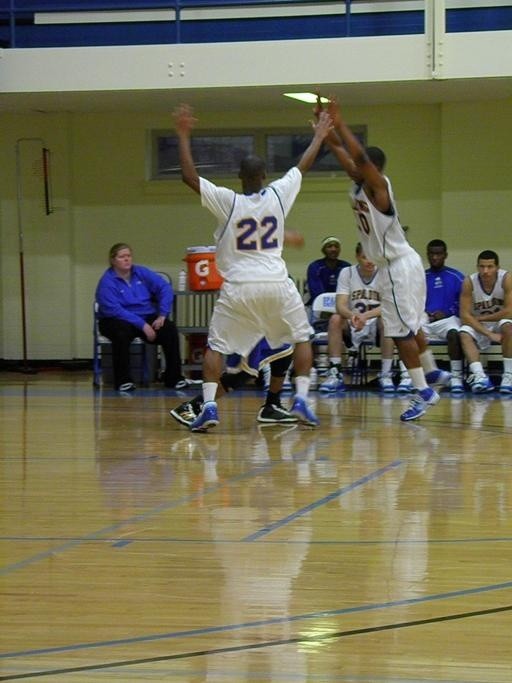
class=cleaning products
[179,269,187,291]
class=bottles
[177,268,186,292]
[310,367,318,390]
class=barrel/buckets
[184,244,223,291]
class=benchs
[314,331,500,390]
[92,290,265,390]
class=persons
[318,241,396,393]
[313,92,455,422]
[457,250,511,395]
[306,235,362,377]
[169,102,335,434]
[263,363,293,393]
[169,230,305,427]
[396,239,466,393]
[95,243,189,392]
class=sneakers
[318,367,346,392]
[164,374,190,390]
[256,395,320,426]
[170,401,220,433]
[400,388,440,421]
[118,382,137,391]
[379,370,512,392]
